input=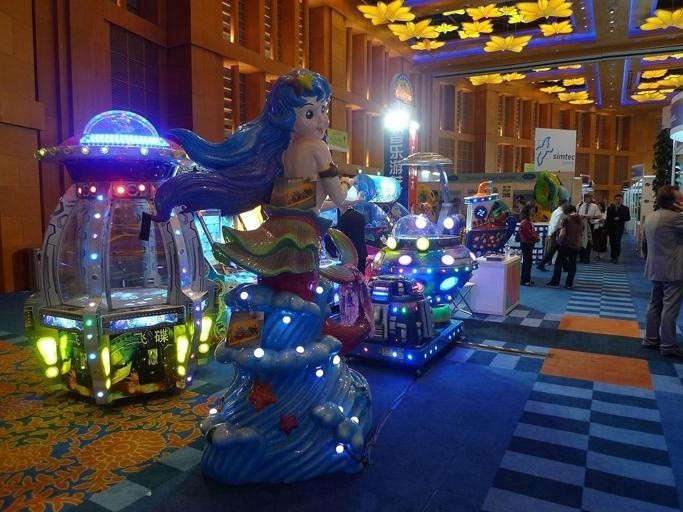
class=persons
[323,209,375,364]
[641,185,682,359]
[153,68,352,300]
[518,194,630,289]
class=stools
[450,281,476,317]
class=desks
[467,255,522,317]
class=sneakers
[545,282,560,289]
[643,339,683,355]
[566,280,573,288]
